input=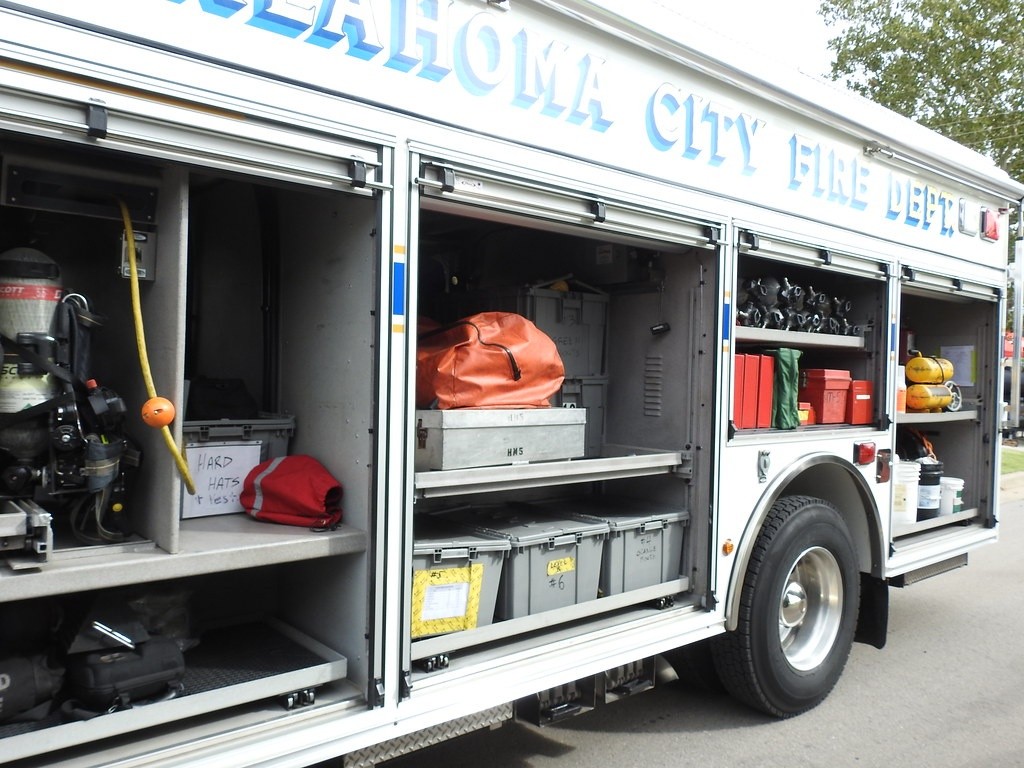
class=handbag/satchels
[416,311,566,409]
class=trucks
[0,0,1024,768]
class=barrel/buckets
[940,478,965,518]
[896,460,921,526]
[916,461,944,522]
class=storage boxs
[415,408,587,471]
[734,352,775,428]
[181,411,296,519]
[491,285,610,457]
[798,369,875,424]
[412,494,691,640]
